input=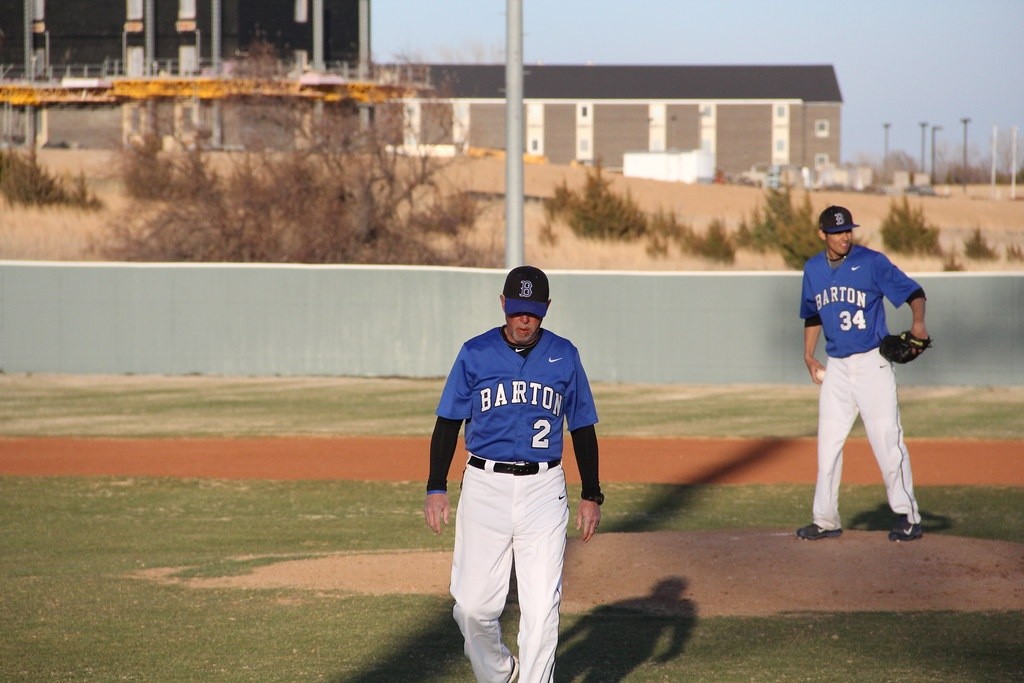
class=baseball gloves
[879,328,932,364]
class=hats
[502,266,549,319]
[819,206,860,233]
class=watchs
[581,493,605,505]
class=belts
[467,456,561,475]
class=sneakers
[796,523,842,541]
[889,514,922,541]
[507,656,518,683]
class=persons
[425,268,600,683]
[797,206,931,542]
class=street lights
[919,122,929,172]
[883,123,890,182]
[960,118,971,183]
[930,126,942,185]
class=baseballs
[815,368,826,382]
